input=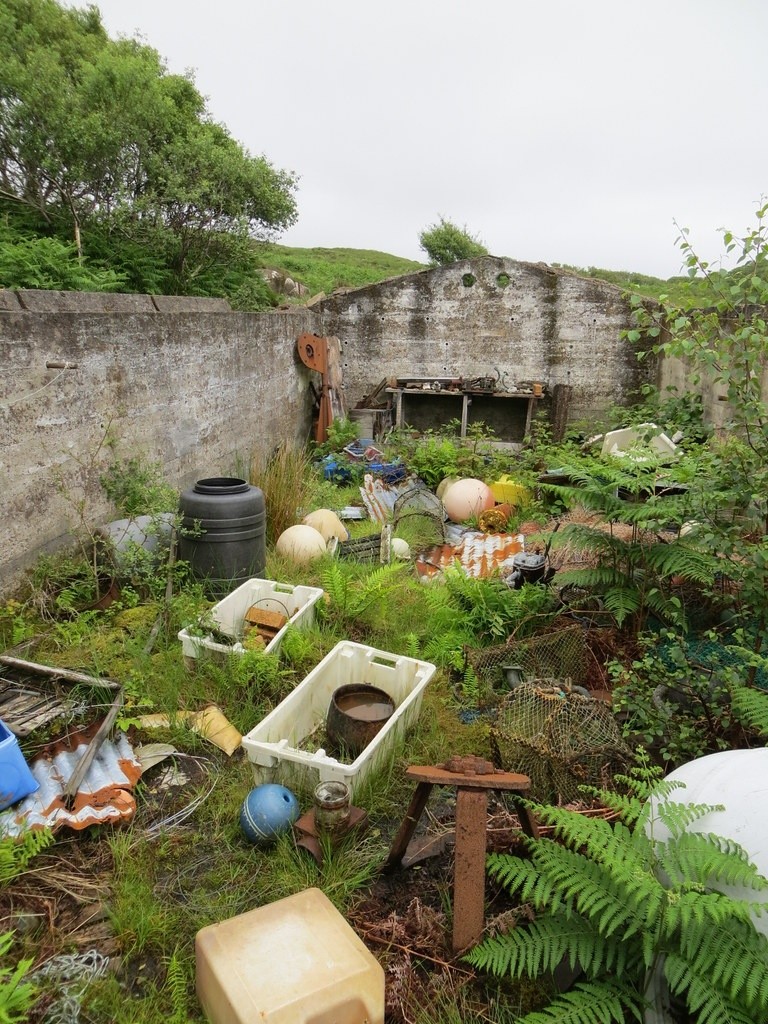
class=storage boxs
[179,578,323,668]
[0,721,40,809]
[602,423,683,460]
[241,639,437,801]
[322,454,403,481]
[195,886,385,1024]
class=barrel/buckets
[327,684,395,755]
[179,478,267,602]
